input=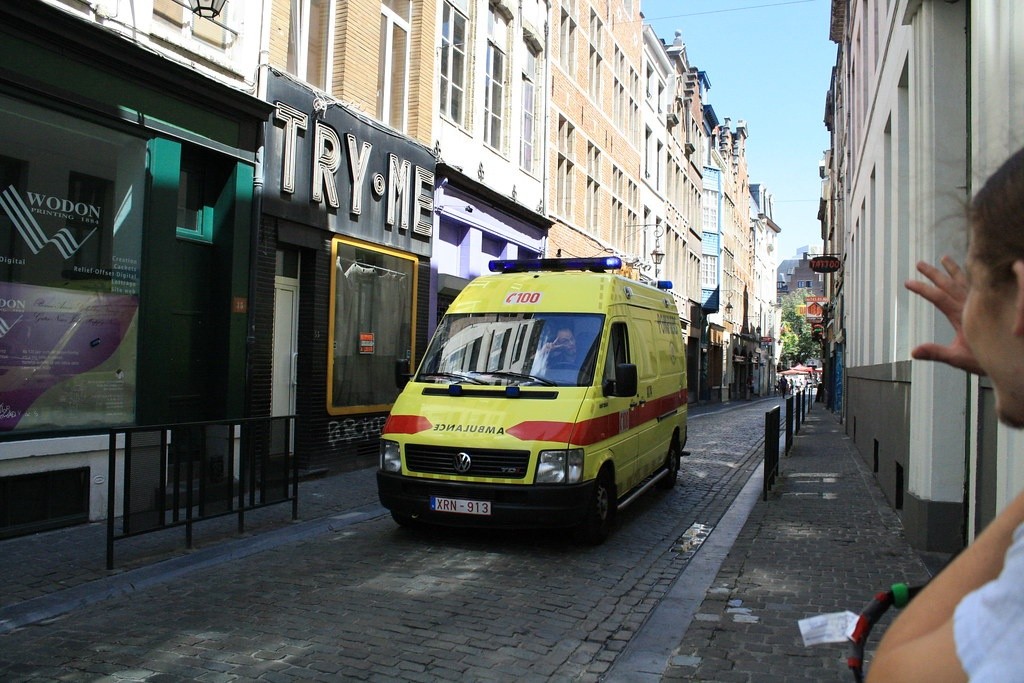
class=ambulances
[376,256,690,545]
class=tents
[777,369,813,390]
[790,363,812,378]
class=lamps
[756,325,761,333]
[622,222,667,266]
[187,0,229,20]
[718,289,736,315]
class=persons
[530,325,589,371]
[774,375,809,398]
[860,146,1023,683]
